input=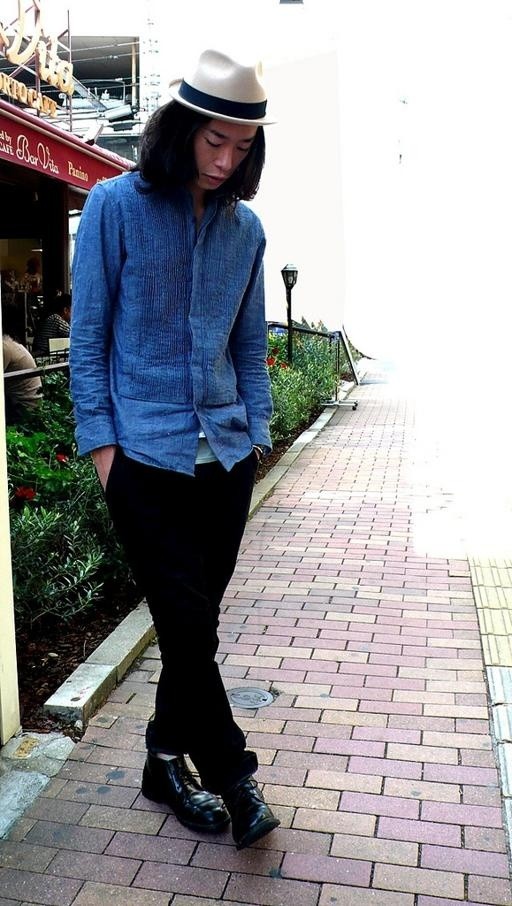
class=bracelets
[252,444,265,465]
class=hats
[167,47,280,127]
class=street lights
[281,264,298,363]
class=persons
[31,293,73,360]
[3,307,47,430]
[67,39,284,855]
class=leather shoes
[140,750,231,833]
[219,774,279,851]
